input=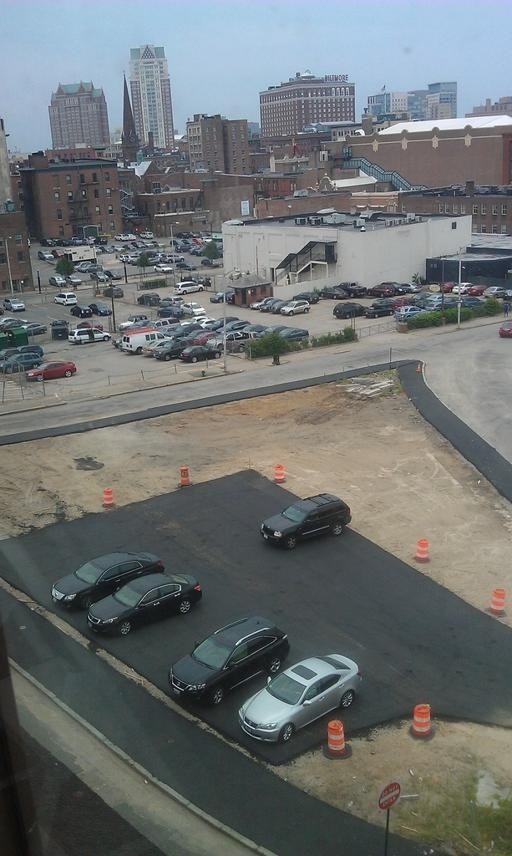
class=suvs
[259,493,350,547]
[169,616,289,705]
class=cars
[104,270,122,280]
[76,319,104,331]
[36,231,108,274]
[111,230,223,270]
[0,317,48,375]
[2,298,26,312]
[112,314,310,363]
[49,319,70,340]
[90,272,109,282]
[279,299,311,316]
[209,289,235,305]
[88,302,112,316]
[24,360,78,382]
[159,296,185,308]
[238,652,362,744]
[70,304,92,319]
[292,291,320,305]
[65,275,81,285]
[137,291,161,307]
[157,306,185,318]
[68,327,112,345]
[87,573,203,636]
[54,292,78,306]
[179,302,206,316]
[102,287,123,298]
[48,276,66,287]
[51,551,164,610]
[173,276,212,296]
[249,296,276,310]
[153,263,173,274]
[319,279,511,322]
[270,300,292,314]
[259,298,284,312]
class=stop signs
[379,783,400,809]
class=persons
[503,302,509,316]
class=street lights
[287,205,292,215]
[170,221,179,288]
[162,201,168,238]
